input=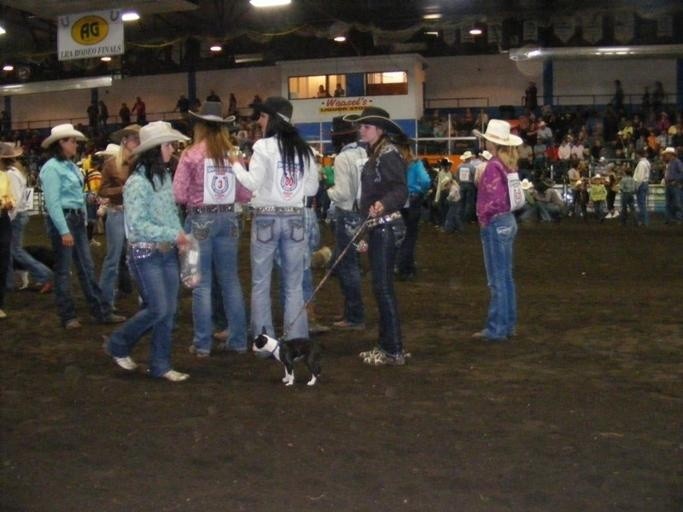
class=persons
[514,78,683,229]
[1,79,523,383]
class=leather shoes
[41,283,52,293]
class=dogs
[311,245,333,272]
[252,326,322,386]
[14,245,56,290]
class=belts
[367,211,401,227]
[258,208,300,213]
[194,205,232,211]
[128,242,169,249]
[63,209,81,213]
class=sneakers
[359,349,404,366]
[187,317,329,359]
[64,319,80,329]
[162,370,189,381]
[112,356,137,371]
[96,314,125,324]
[333,321,364,330]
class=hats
[188,101,234,123]
[520,179,532,189]
[329,116,357,135]
[94,120,191,163]
[342,106,407,144]
[437,150,492,165]
[472,119,523,145]
[249,96,298,132]
[541,177,556,187]
[0,142,22,158]
[40,123,85,149]
[661,147,675,154]
